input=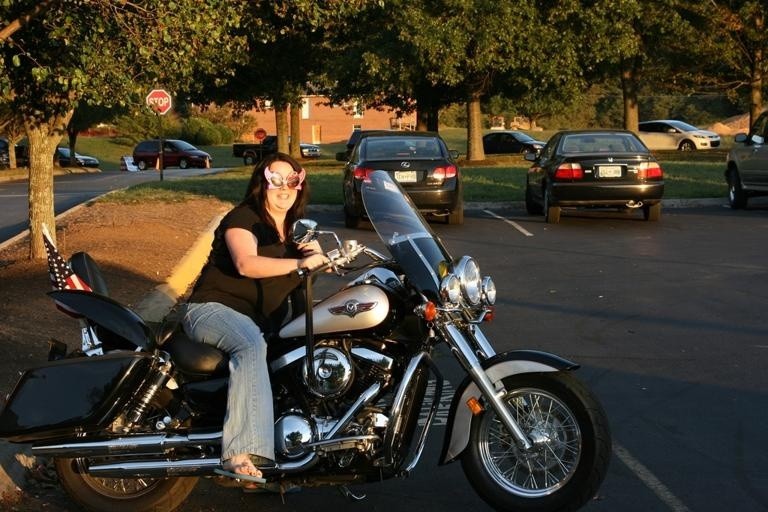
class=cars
[0,138,26,170]
[345,129,408,163]
[724,107,768,210]
[336,131,464,229]
[131,139,212,171]
[22,147,100,168]
[524,129,664,225]
[637,120,720,152]
[482,131,548,154]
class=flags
[39,222,94,320]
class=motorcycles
[0,168,612,511]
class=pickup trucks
[232,135,322,167]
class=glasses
[264,166,305,190]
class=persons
[181,152,333,496]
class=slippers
[214,461,266,483]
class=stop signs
[254,128,267,141]
[146,89,171,115]
